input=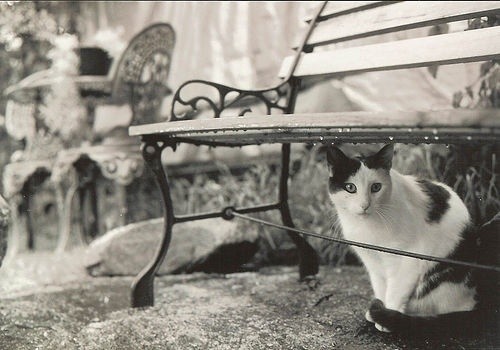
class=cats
[327,142,488,338]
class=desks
[4,76,116,239]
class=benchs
[129,0,498,307]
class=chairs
[53,26,174,253]
